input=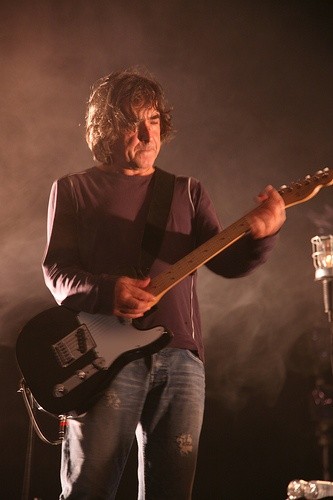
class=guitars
[15,168,333,416]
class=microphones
[311,235,333,313]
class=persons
[42,71,286,500]
